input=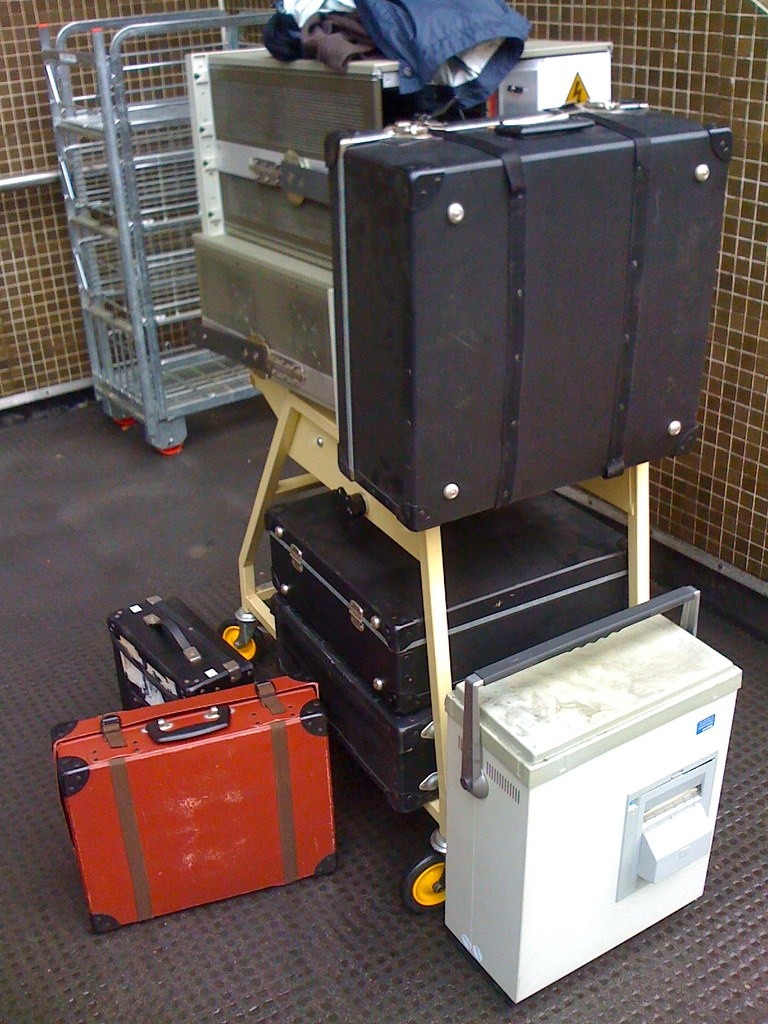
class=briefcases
[50,676,338,935]
[187,48,744,813]
[107,593,254,710]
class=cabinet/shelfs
[36,8,277,454]
